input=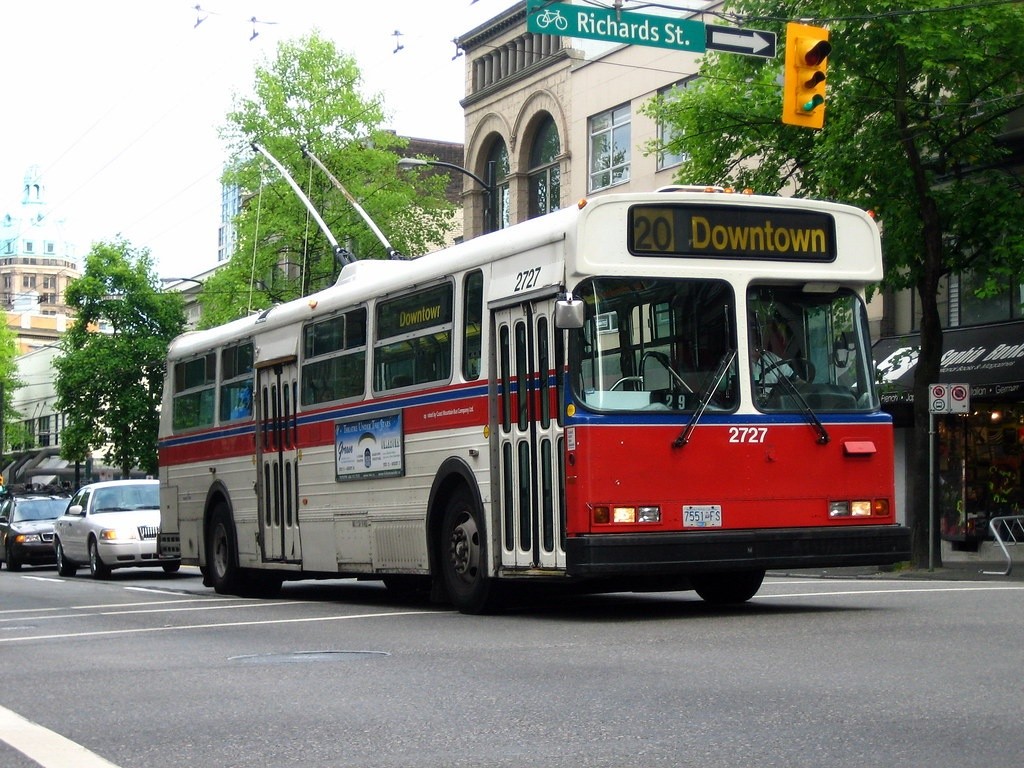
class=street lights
[399,157,496,234]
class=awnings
[826,319,1024,406]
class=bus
[157,144,940,617]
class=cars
[53,479,181,580]
[0,492,76,572]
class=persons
[315,386,335,402]
[716,309,807,408]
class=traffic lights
[781,23,833,130]
